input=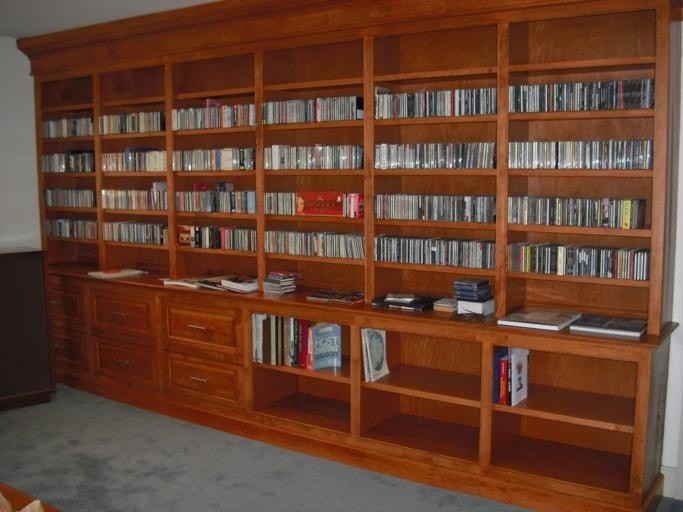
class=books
[99,110,168,247]
[374,86,497,271]
[171,96,257,253]
[433,295,458,313]
[496,306,582,332]
[262,269,301,295]
[569,310,647,338]
[261,93,364,262]
[493,346,529,407]
[305,288,365,306]
[38,115,98,240]
[453,278,491,301]
[87,268,147,279]
[163,274,258,293]
[371,288,437,312]
[508,73,654,281]
[360,327,391,383]
[251,311,342,370]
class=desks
[0,481,63,511]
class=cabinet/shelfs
[1,246,54,411]
[16,0,683,510]
